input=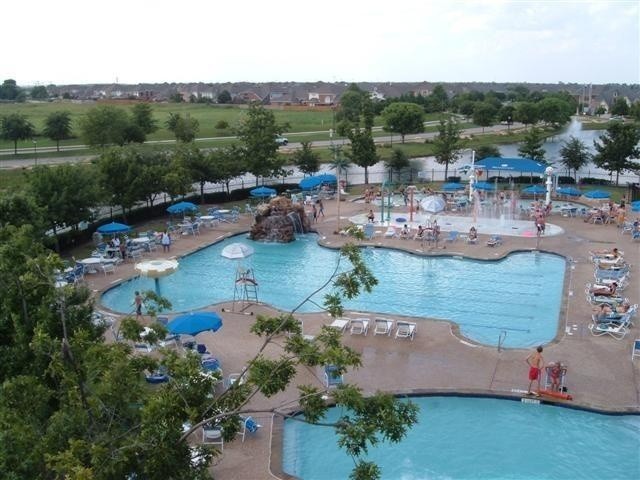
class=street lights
[545,166,554,206]
[506,113,514,133]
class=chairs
[321,362,345,392]
[83,307,263,453]
[56,188,339,287]
[339,187,640,253]
[287,318,305,343]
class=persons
[312,204,318,224]
[160,229,171,252]
[107,236,130,261]
[362,182,552,246]
[544,361,567,392]
[129,291,146,322]
[316,199,324,217]
[588,247,630,324]
[523,346,545,397]
[583,194,628,225]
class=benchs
[583,244,640,361]
[327,313,419,342]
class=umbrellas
[583,189,610,201]
[96,222,130,240]
[220,242,254,261]
[249,186,277,204]
[165,201,197,214]
[297,174,337,190]
[165,310,224,337]
[555,186,582,198]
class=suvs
[262,132,290,146]
